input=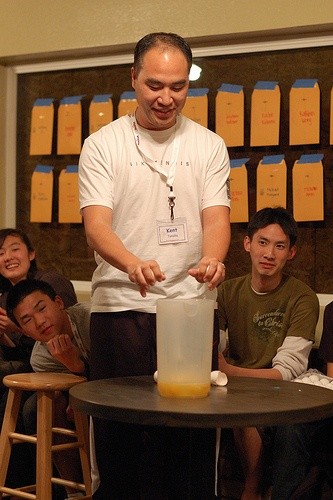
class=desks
[69,374,333,500]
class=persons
[218,206,332,500]
[78,32,231,500]
[0,205,90,500]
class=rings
[222,263,226,268]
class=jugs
[154,298,214,399]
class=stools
[0,373,91,500]
